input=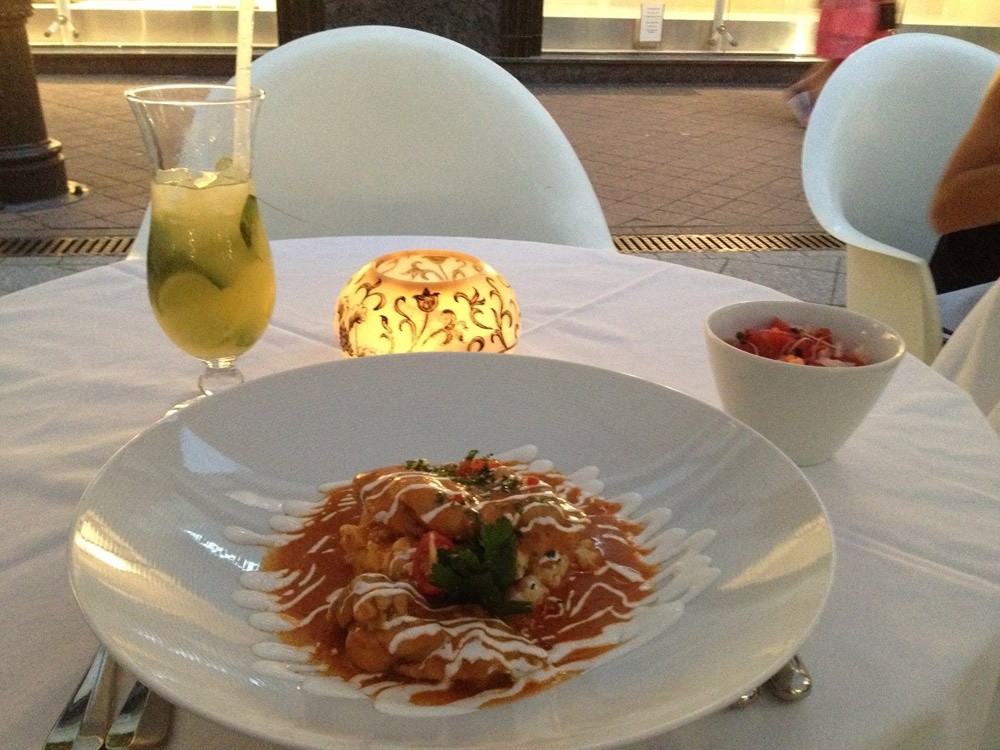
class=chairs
[124,26,616,258]
[801,33,1000,364]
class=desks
[0,235,1000,749]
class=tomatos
[735,317,861,367]
[441,490,465,503]
[414,531,455,594]
[456,457,502,477]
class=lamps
[332,248,522,356]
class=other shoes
[785,90,815,128]
[785,78,805,97]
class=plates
[70,353,839,750]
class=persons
[930,68,1000,432]
[787,0,897,129]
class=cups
[704,300,905,467]
[335,247,520,352]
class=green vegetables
[148,195,259,348]
[405,450,556,615]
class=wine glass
[125,83,277,422]
